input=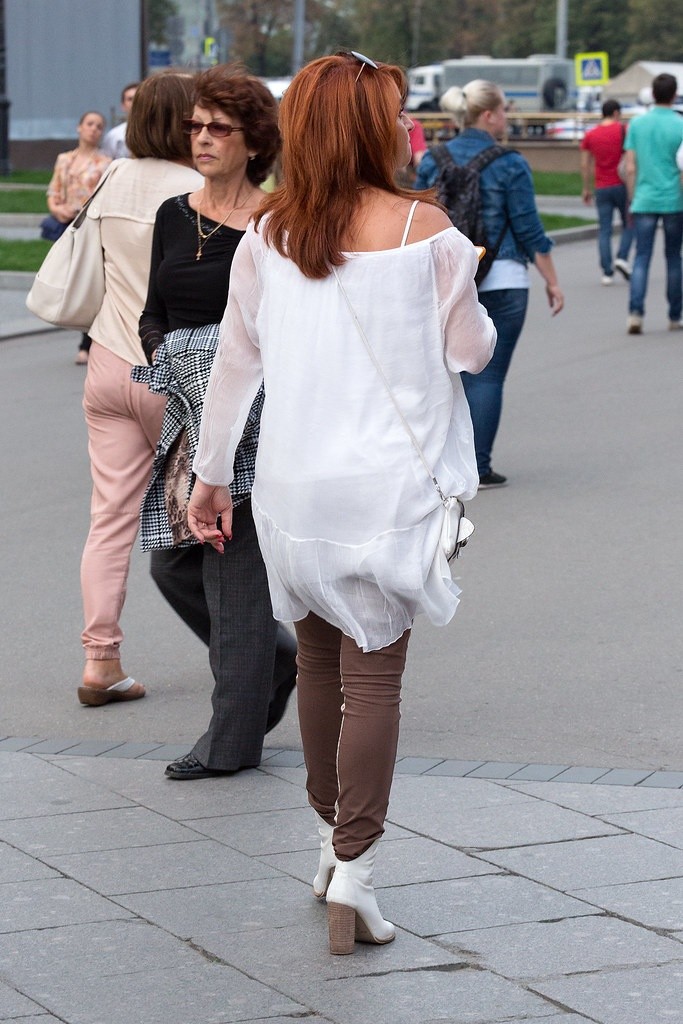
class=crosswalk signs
[581,59,603,80]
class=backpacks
[429,143,520,290]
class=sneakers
[479,467,510,489]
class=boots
[326,828,396,954]
[312,806,338,898]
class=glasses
[179,118,245,138]
[335,49,379,84]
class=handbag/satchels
[441,496,475,567]
[24,157,127,333]
[39,154,75,241]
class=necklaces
[196,192,254,261]
[356,186,365,189]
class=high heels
[77,676,145,703]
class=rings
[203,523,207,526]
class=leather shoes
[165,753,240,779]
[265,670,297,733]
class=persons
[414,80,565,490]
[418,97,439,111]
[580,101,632,285]
[409,122,428,165]
[137,70,297,778]
[78,70,205,708]
[623,73,683,333]
[47,111,115,362]
[188,51,501,955]
[103,82,140,158]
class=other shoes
[615,258,632,280]
[670,321,683,330]
[626,313,642,333]
[601,276,613,284]
[75,350,89,364]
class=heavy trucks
[403,53,578,136]
